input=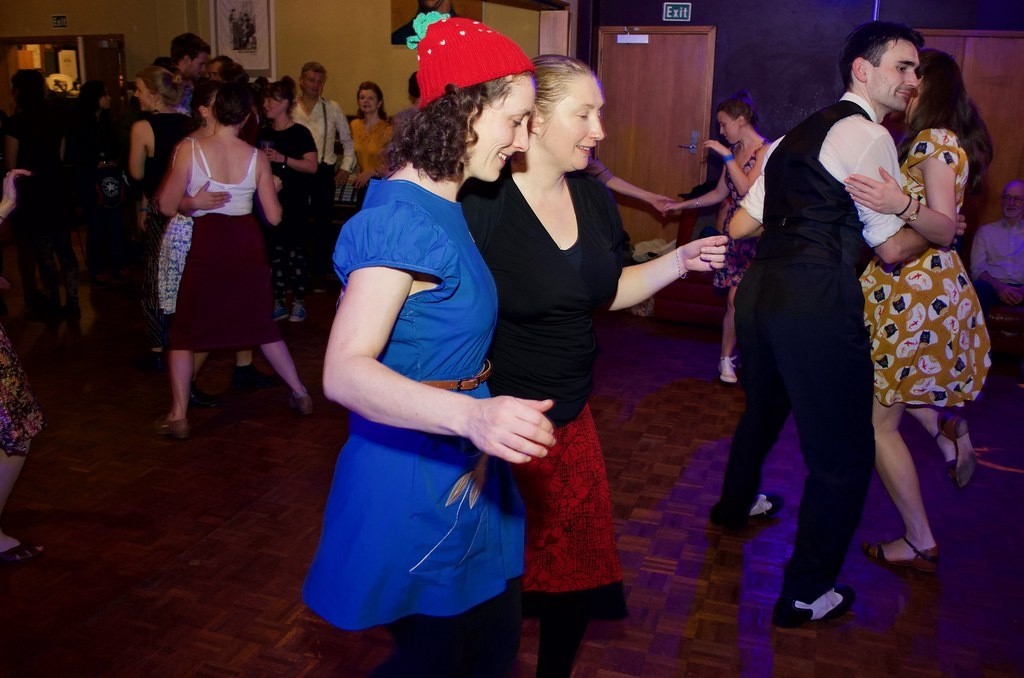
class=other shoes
[286,388,313,414]
[0,541,47,563]
[153,415,190,438]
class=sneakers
[288,301,308,321]
[773,586,856,627]
[272,303,290,322]
[711,490,785,524]
[718,356,738,383]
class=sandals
[935,410,977,488]
[862,536,939,574]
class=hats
[406,10,535,111]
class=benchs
[650,205,1022,364]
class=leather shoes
[133,351,164,371]
[189,380,223,406]
[231,362,276,387]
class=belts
[420,358,492,390]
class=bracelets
[677,250,687,279]
[696,198,698,208]
[284,155,288,164]
[0,216,5,220]
[375,168,377,176]
[896,194,912,216]
[721,155,734,163]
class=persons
[259,76,318,323]
[302,12,556,678]
[349,82,393,189]
[207,56,233,82]
[457,53,726,678]
[586,157,675,216]
[713,21,925,626]
[151,78,313,440]
[291,62,356,280]
[164,32,209,111]
[860,48,990,578]
[0,57,198,372]
[660,89,770,384]
[970,179,1024,386]
[0,170,46,562]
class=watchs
[904,201,920,222]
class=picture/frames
[209,0,277,83]
